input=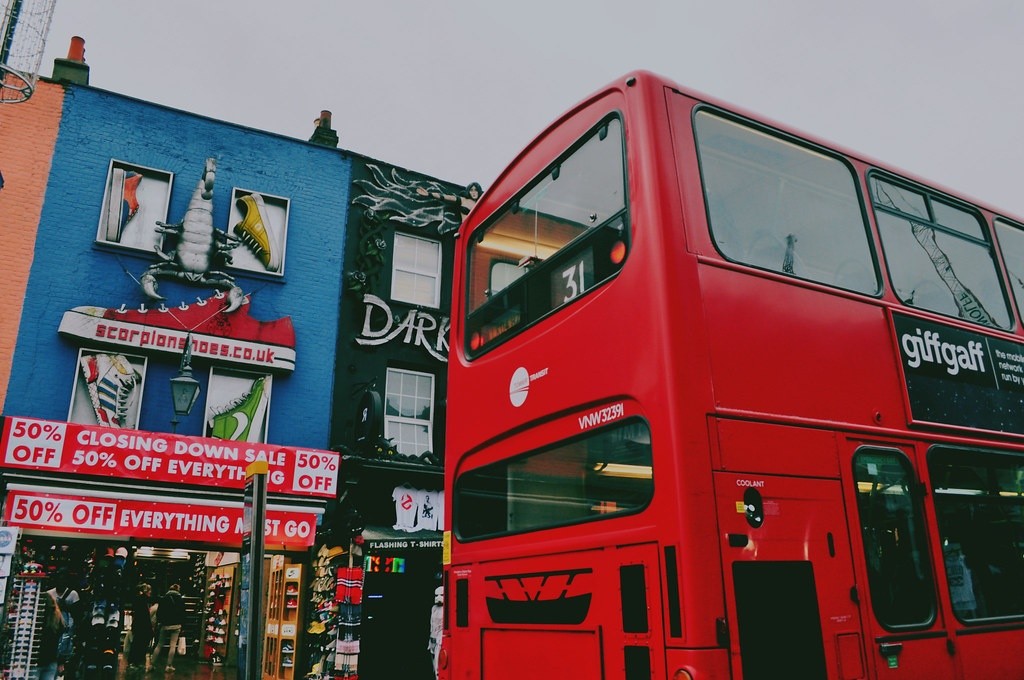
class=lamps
[170,333,200,416]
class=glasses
[3,577,40,680]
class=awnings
[361,526,444,549]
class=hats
[87,546,128,669]
[327,546,348,561]
[307,663,321,675]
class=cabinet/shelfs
[262,558,308,680]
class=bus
[433,66,1024,680]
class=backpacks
[44,589,69,633]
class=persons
[125,584,153,670]
[429,586,444,680]
[148,585,185,673]
[29,570,83,680]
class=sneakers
[287,598,297,608]
[282,657,293,667]
[282,644,294,653]
[208,377,270,444]
[286,586,298,595]
[107,168,143,243]
[233,194,279,273]
[81,354,142,429]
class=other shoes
[165,665,175,672]
[203,573,232,666]
[145,665,157,671]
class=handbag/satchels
[56,627,73,659]
[146,641,153,653]
[177,637,187,655]
